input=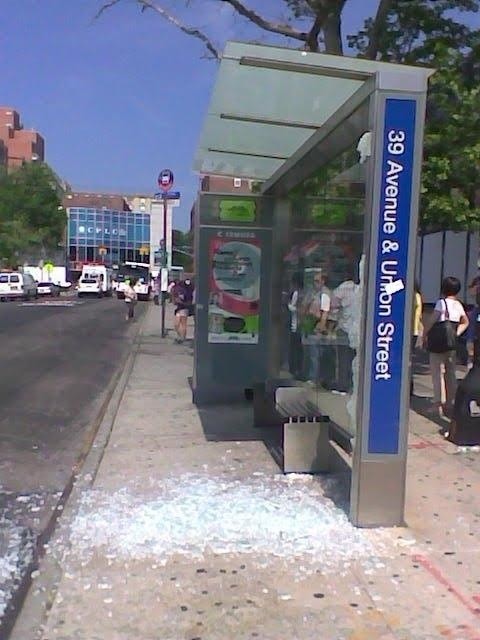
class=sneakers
[332,390,346,396]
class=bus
[112,260,153,301]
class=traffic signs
[153,190,183,200]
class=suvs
[78,278,104,299]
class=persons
[422,277,470,416]
[277,262,364,398]
[409,276,426,400]
[171,280,191,344]
[437,276,480,441]
[123,281,138,321]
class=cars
[35,281,62,297]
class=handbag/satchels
[425,319,457,354]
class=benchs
[246,374,332,479]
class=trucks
[1,271,39,302]
[80,264,113,298]
[17,264,68,285]
